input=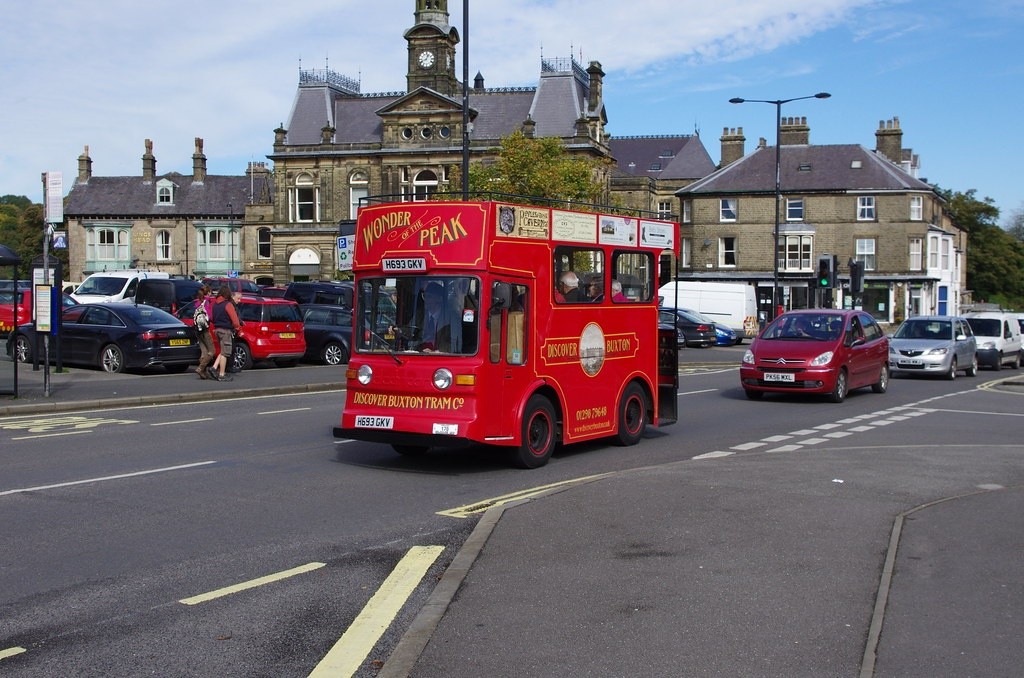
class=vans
[64,269,175,306]
[135,279,209,315]
[654,281,757,344]
[960,312,1021,371]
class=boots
[199,357,212,379]
[195,354,211,379]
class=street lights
[226,202,234,270]
[729,92,831,338]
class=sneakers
[208,367,218,379]
[217,373,233,381]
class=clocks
[445,51,452,71]
[416,49,437,71]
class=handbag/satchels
[193,299,210,332]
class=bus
[333,192,680,470]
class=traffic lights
[820,259,830,288]
[833,255,840,288]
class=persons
[826,320,866,347]
[909,326,925,338]
[449,271,633,325]
[194,285,246,382]
[386,282,462,353]
[782,319,816,338]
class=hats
[424,282,444,297]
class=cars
[740,309,889,403]
[658,307,717,348]
[678,308,736,346]
[171,295,306,373]
[298,304,395,365]
[889,315,978,380]
[0,288,85,337]
[6,303,202,373]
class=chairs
[585,272,644,303]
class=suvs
[261,279,396,324]
[199,277,261,297]
